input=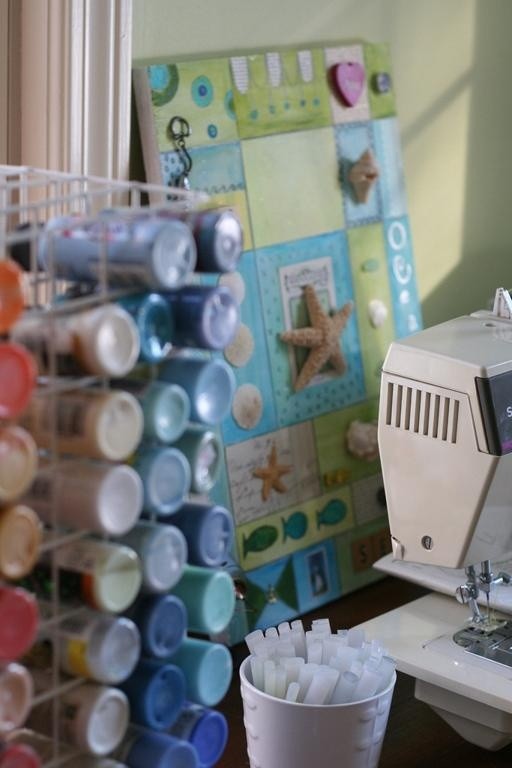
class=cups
[240,649,397,768]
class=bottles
[0,205,244,768]
